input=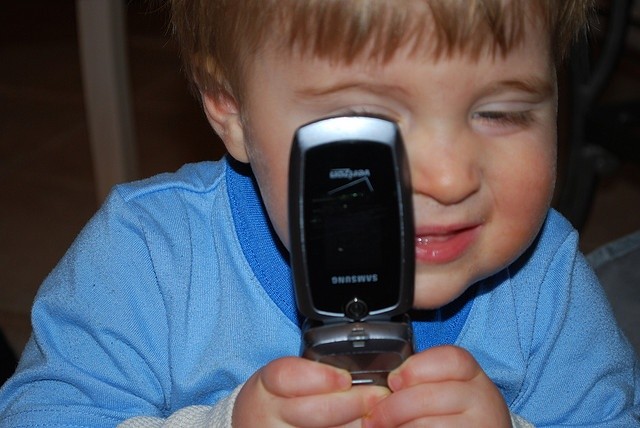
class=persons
[1,0,640,428]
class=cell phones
[286,115,416,391]
